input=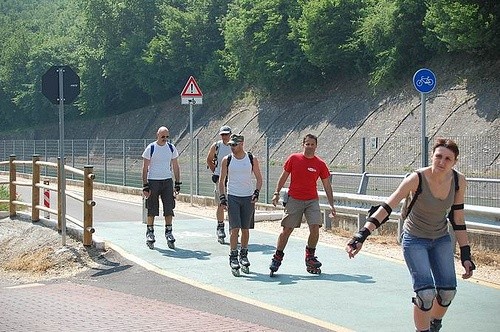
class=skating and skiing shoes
[145,225,155,249]
[217,222,226,244]
[228,249,241,277]
[164,225,177,249]
[304,245,323,274]
[269,249,285,276]
[238,247,251,275]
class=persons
[345,139,475,332]
[269,135,336,277]
[207,126,233,244]
[142,126,183,249]
[217,134,262,278]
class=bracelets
[274,192,279,195]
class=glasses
[229,143,238,146]
[161,136,169,139]
[220,134,228,136]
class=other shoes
[431,318,443,332]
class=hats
[219,126,232,136]
[227,133,244,144]
